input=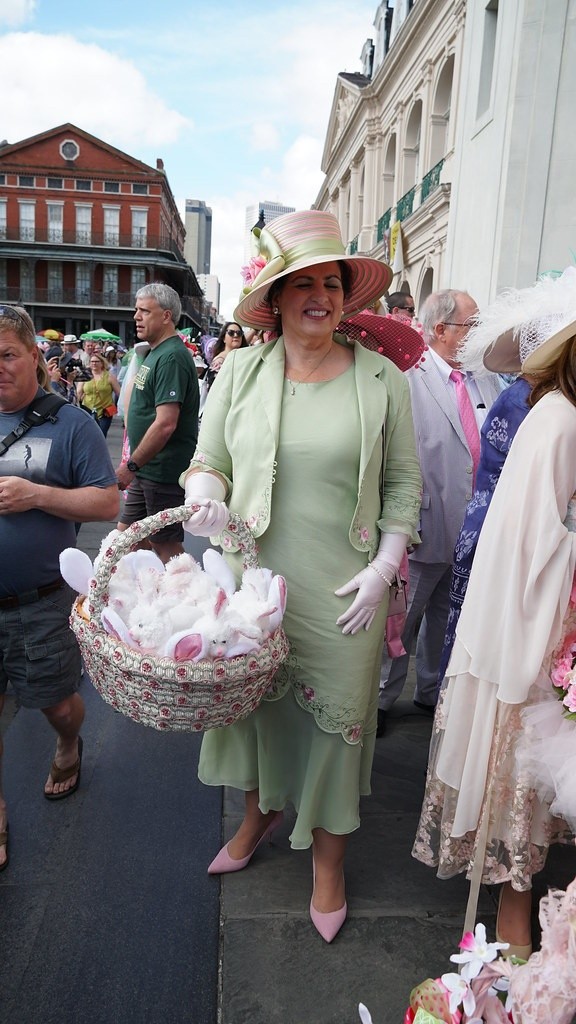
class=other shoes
[414,694,437,713]
[493,882,533,967]
[375,707,386,738]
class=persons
[420,319,575,959]
[179,216,420,942]
[0,301,120,862]
[357,282,506,737]
[116,282,201,565]
[24,323,267,440]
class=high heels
[310,848,347,944]
[208,805,283,874]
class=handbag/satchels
[104,406,118,418]
[387,571,407,616]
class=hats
[464,281,547,375]
[44,328,59,339]
[521,269,576,384]
[60,334,81,344]
[345,307,428,373]
[232,210,396,330]
[192,356,208,369]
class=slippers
[43,735,82,798]
[0,819,10,871]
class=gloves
[181,471,229,536]
[334,533,408,635]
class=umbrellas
[79,327,122,344]
[37,328,64,340]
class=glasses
[393,305,415,314]
[225,329,245,337]
[91,360,100,363]
[0,305,33,335]
[441,321,480,330]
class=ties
[450,369,481,498]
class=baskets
[69,503,289,734]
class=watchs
[127,459,139,472]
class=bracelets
[368,563,392,586]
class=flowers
[549,638,576,713]
[240,256,267,286]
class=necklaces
[283,343,334,395]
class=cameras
[66,358,82,373]
